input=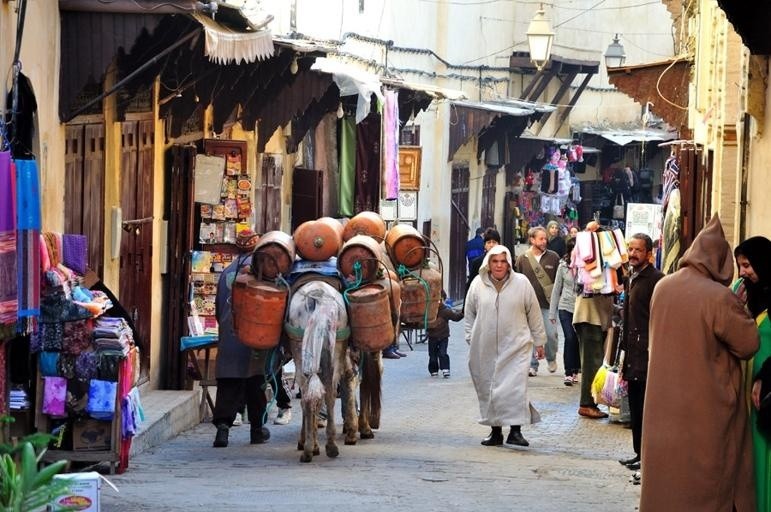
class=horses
[280,281,382,465]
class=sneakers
[528,368,538,377]
[546,359,558,373]
[480,432,505,447]
[382,347,408,361]
[431,371,438,378]
[506,430,530,447]
[563,369,581,387]
[211,423,230,448]
[250,426,270,444]
[442,368,451,377]
[273,406,292,426]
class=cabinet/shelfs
[28,344,139,478]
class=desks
[182,333,224,424]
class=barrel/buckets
[238,279,288,350]
[337,235,381,282]
[295,216,343,261]
[230,273,257,337]
[374,277,401,337]
[384,223,425,271]
[382,268,398,282]
[345,279,402,354]
[410,267,443,325]
[342,210,386,243]
[399,278,433,325]
[250,230,293,283]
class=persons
[515,221,610,419]
[464,245,547,447]
[428,224,515,376]
[383,348,407,359]
[640,212,771,511]
[211,229,302,448]
[618,233,665,469]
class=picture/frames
[395,144,422,192]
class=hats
[235,229,261,252]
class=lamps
[604,30,630,68]
[335,100,345,120]
[525,1,555,73]
[287,54,300,75]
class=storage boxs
[71,417,112,452]
[46,469,119,512]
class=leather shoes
[577,406,609,419]
